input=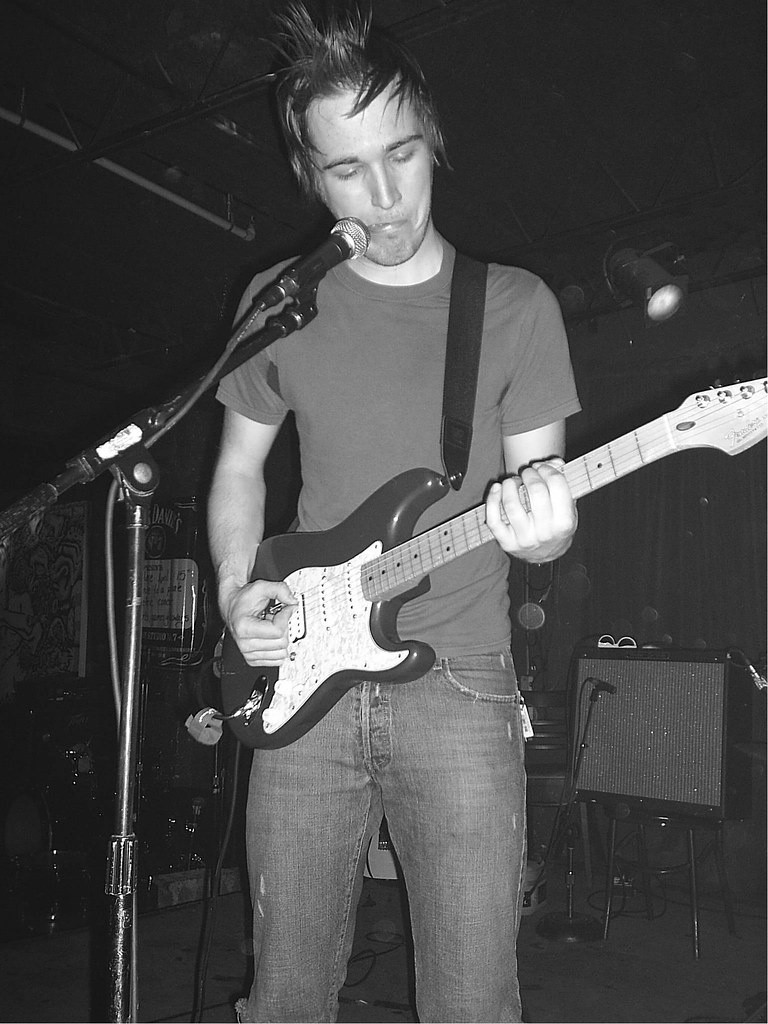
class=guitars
[219,377,768,751]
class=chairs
[515,690,596,901]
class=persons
[203,8,580,1024]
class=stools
[597,808,738,962]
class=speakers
[0,657,255,941]
[570,646,758,823]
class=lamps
[610,246,682,322]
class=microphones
[588,676,619,696]
[581,795,631,820]
[252,215,374,315]
